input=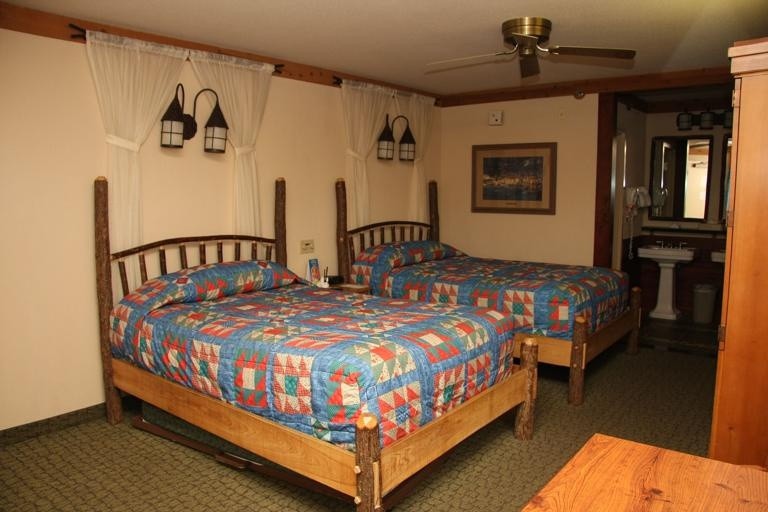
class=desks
[521,433,768,512]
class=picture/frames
[471,142,557,216]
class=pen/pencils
[324,266,328,282]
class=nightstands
[326,283,370,295]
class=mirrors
[647,135,713,223]
[718,134,732,221]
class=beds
[336,179,643,406]
[94,176,538,512]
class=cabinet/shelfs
[705,36,768,468]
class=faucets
[667,240,673,248]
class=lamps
[677,105,732,130]
[377,113,416,161]
[160,84,229,153]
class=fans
[422,17,636,80]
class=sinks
[638,242,695,263]
[710,248,725,263]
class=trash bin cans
[692,284,718,325]
